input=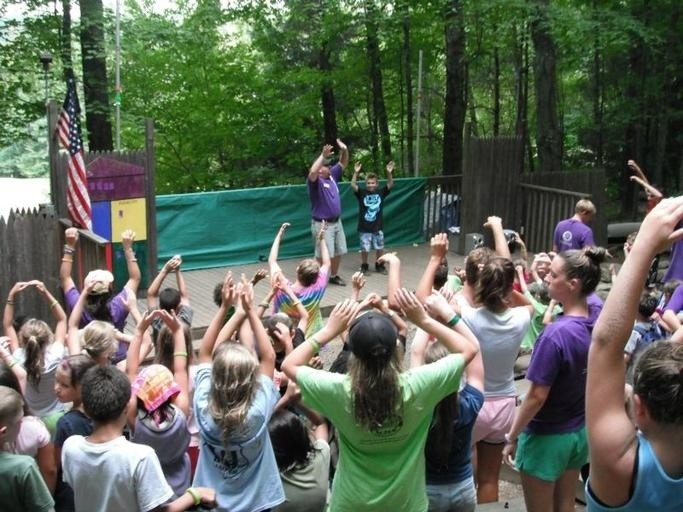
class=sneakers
[361,264,369,274]
[329,276,346,287]
[376,262,388,275]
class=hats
[85,269,114,296]
[349,313,398,360]
[502,228,516,245]
[310,157,335,166]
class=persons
[583,194,680,511]
[502,245,604,512]
[1,406,56,510]
[552,199,596,253]
[416,232,533,504]
[515,263,563,357]
[648,186,683,314]
[622,294,669,378]
[57,227,139,327]
[212,270,268,332]
[350,271,368,305]
[60,365,216,511]
[408,280,483,511]
[69,278,137,378]
[0,386,57,493]
[482,215,530,289]
[147,254,192,364]
[256,271,308,370]
[350,160,394,273]
[271,322,313,377]
[53,357,95,511]
[265,381,333,510]
[267,218,331,360]
[512,232,552,287]
[307,138,348,286]
[376,253,446,311]
[125,310,190,508]
[0,334,28,392]
[211,286,256,361]
[192,269,282,512]
[630,159,664,291]
[2,280,64,414]
[277,288,475,510]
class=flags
[51,68,96,234]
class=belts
[312,216,340,223]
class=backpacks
[636,321,664,345]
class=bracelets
[123,248,134,253]
[61,258,72,262]
[3,298,17,306]
[4,359,18,369]
[63,244,73,254]
[46,300,58,309]
[171,352,188,359]
[386,311,396,316]
[186,483,197,506]
[309,338,324,352]
[126,258,138,262]
[258,301,271,311]
[502,432,516,442]
[443,313,464,330]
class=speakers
[502,229,515,254]
[464,233,484,256]
